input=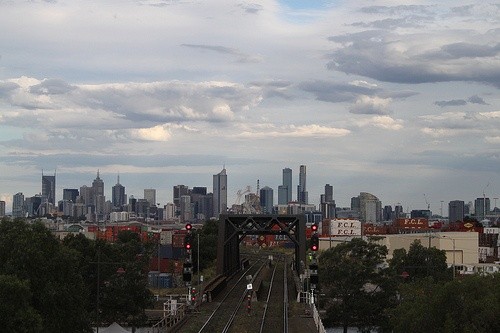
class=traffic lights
[310,225,318,231]
[186,243,191,249]
[310,235,319,252]
[185,224,192,230]
